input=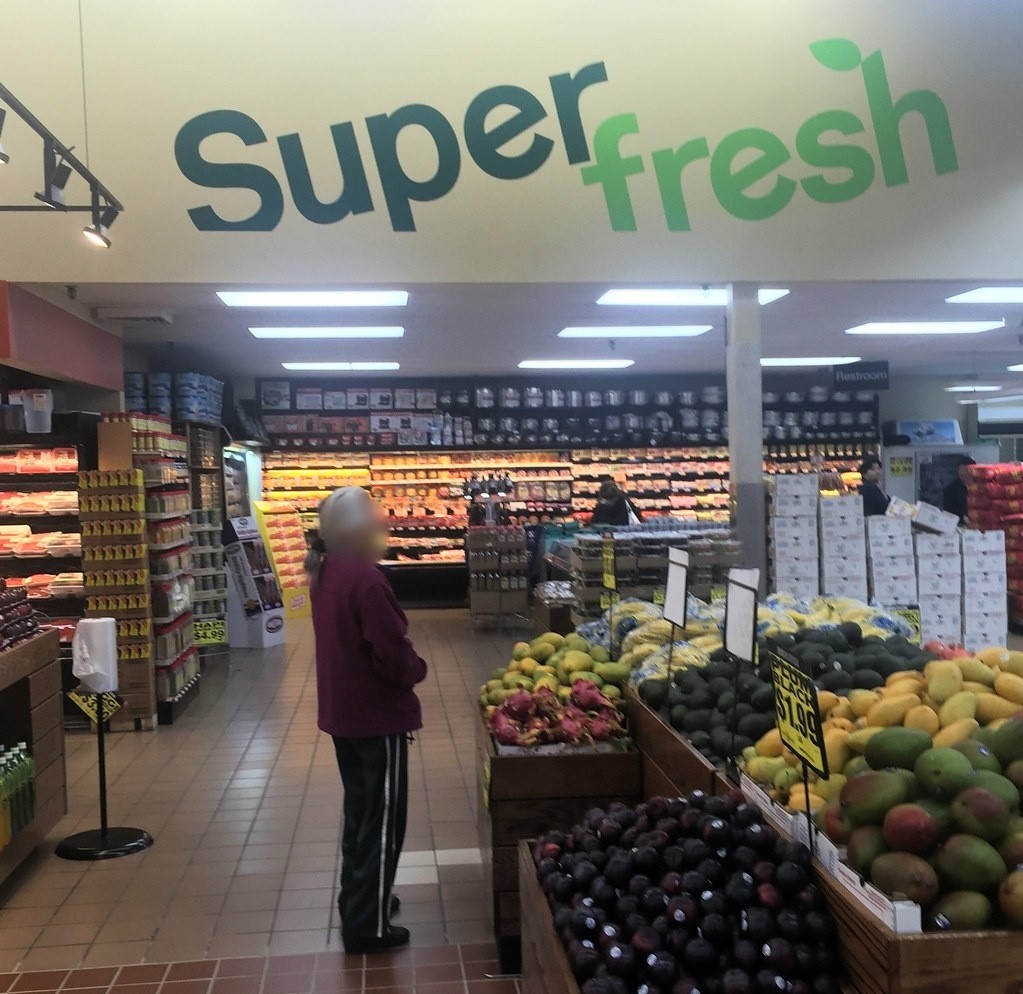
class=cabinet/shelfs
[255,370,880,453]
[77,413,231,734]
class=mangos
[747,647,1023,818]
[811,707,1023,930]
[478,632,631,714]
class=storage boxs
[415,389,437,409]
[767,496,817,517]
[473,697,923,994]
[345,388,370,409]
[819,577,869,598]
[819,539,866,557]
[769,474,819,496]
[767,579,819,600]
[766,516,817,538]
[817,495,865,517]
[324,390,346,409]
[393,389,416,409]
[369,388,393,409]
[767,560,818,579]
[766,538,818,560]
[568,546,747,625]
[819,558,867,578]
[865,495,1008,653]
[817,516,864,540]
[467,533,528,614]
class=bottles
[101,411,226,699]
[463,470,513,493]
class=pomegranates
[922,640,973,660]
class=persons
[590,476,643,527]
[860,459,890,516]
[946,457,978,524]
[305,486,430,950]
[468,487,509,526]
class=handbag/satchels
[625,501,641,526]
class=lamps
[34,162,73,213]
[0,107,9,164]
[82,205,119,249]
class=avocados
[638,621,938,771]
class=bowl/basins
[1,490,83,599]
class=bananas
[604,597,895,681]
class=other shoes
[389,894,403,914]
[344,925,413,954]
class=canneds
[100,411,187,452]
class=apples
[0,577,38,647]
